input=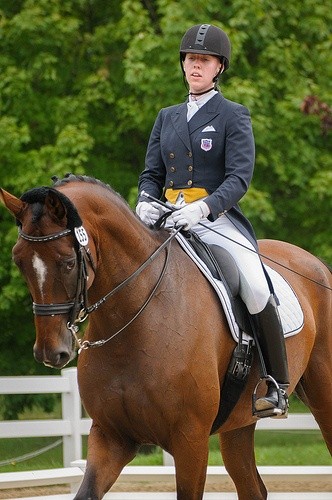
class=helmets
[179,24,231,75]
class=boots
[246,294,290,419]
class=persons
[135,24,290,419]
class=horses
[0,174,332,500]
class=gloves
[172,201,210,231]
[136,201,167,229]
